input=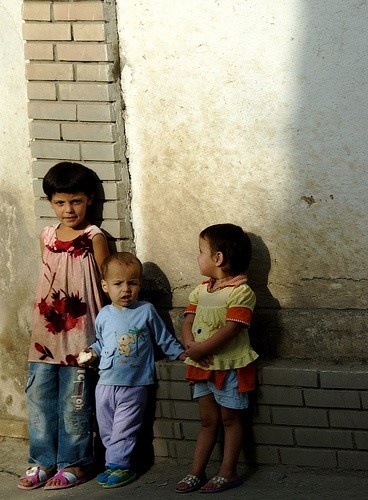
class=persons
[176,224,259,492]
[17,160,109,490]
[77,252,186,487]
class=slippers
[199,475,242,493]
[17,464,56,490]
[43,470,88,490]
[174,474,209,493]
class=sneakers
[97,468,136,488]
